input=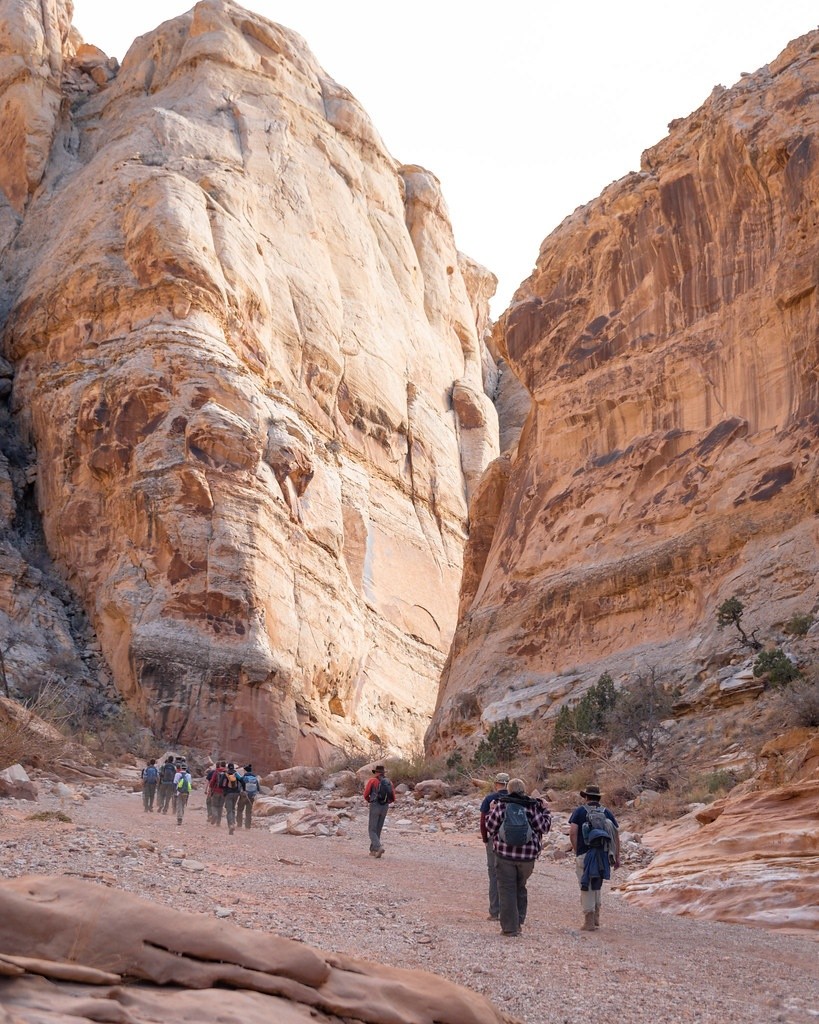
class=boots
[580,911,595,930]
[594,903,601,926]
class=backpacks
[498,801,532,845]
[226,773,238,789]
[582,804,607,849]
[246,779,257,799]
[163,763,175,782]
[217,770,228,787]
[378,777,390,805]
[146,767,157,784]
[176,774,191,793]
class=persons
[486,778,552,938]
[236,764,260,830]
[480,773,510,921]
[568,784,619,931]
[363,766,396,858]
[173,764,191,825]
[141,756,191,815]
[204,762,228,826]
[221,763,246,835]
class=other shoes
[375,846,385,858]
[487,916,500,921]
[369,851,375,856]
[143,805,182,825]
[207,816,251,835]
[501,928,522,937]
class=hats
[494,773,509,783]
[372,766,388,774]
[175,764,180,770]
[181,764,186,768]
[580,785,604,798]
[243,764,252,772]
[175,756,182,763]
[228,764,235,769]
